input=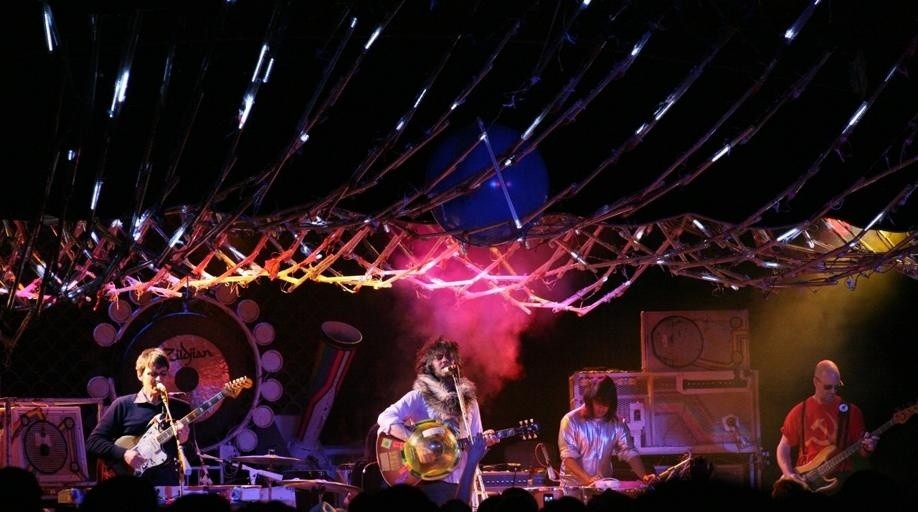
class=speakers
[640,309,751,372]
[649,371,762,446]
[9,406,89,483]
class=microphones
[157,383,166,392]
[443,366,452,373]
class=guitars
[366,418,542,487]
[771,403,918,497]
[112,376,254,479]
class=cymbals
[233,455,305,466]
[279,479,363,494]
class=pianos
[564,478,652,495]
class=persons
[87,348,197,482]
[770,467,916,511]
[543,466,769,512]
[80,474,159,510]
[557,375,661,501]
[1,467,43,511]
[777,359,878,491]
[165,493,297,511]
[377,334,500,510]
[347,483,539,511]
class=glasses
[814,375,844,390]
[433,349,453,361]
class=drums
[153,484,297,509]
